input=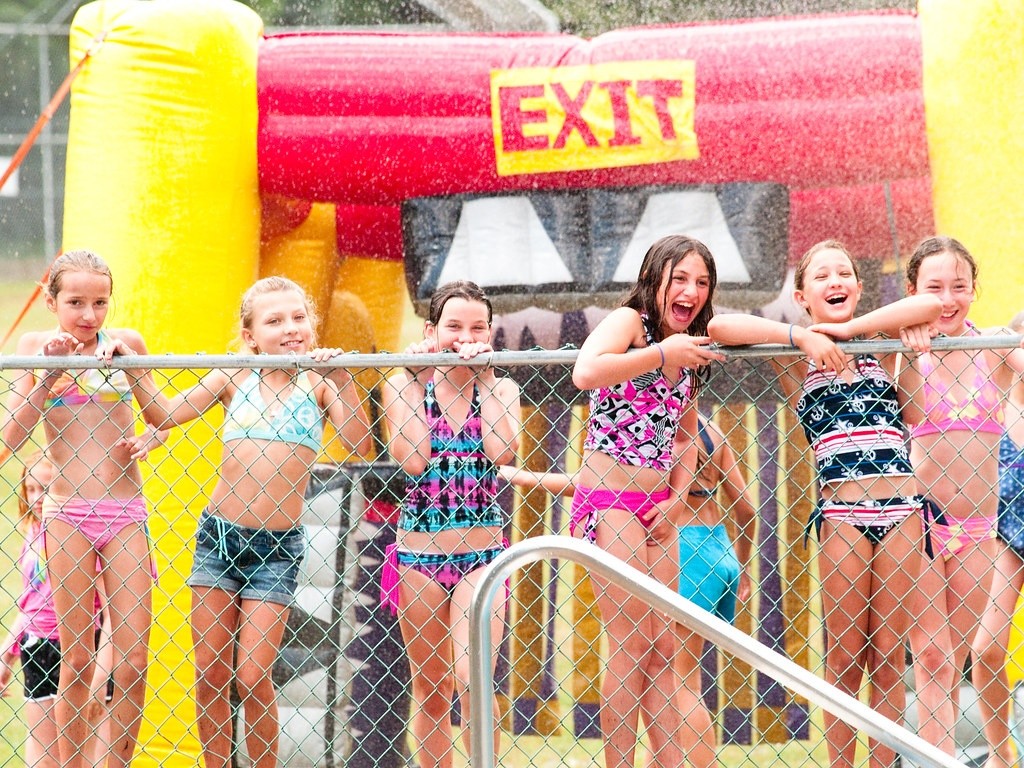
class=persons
[0,252,171,768]
[501,235,1024,768]
[384,278,520,767]
[95,276,371,768]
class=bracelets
[790,324,795,349]
[656,345,665,367]
